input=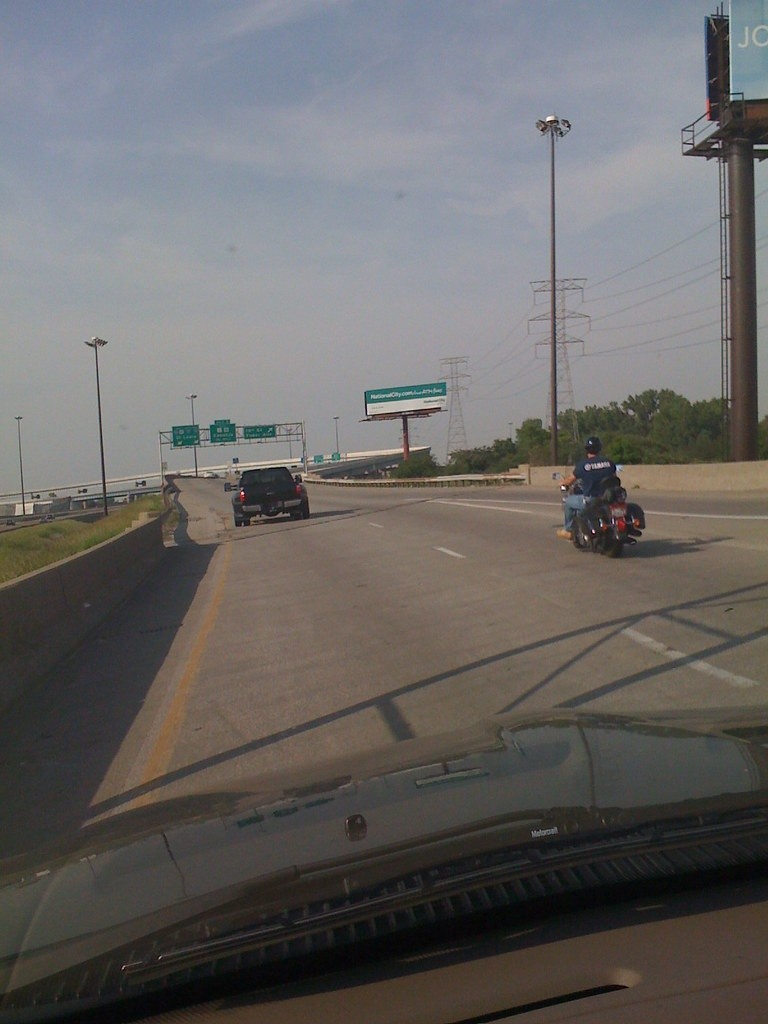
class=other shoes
[557,529,572,541]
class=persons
[556,437,617,541]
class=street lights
[185,394,198,477]
[14,416,26,517]
[333,416,340,454]
[534,113,573,467]
[84,336,110,520]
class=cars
[224,466,310,528]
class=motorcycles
[552,465,646,558]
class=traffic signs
[244,425,277,439]
[172,425,200,447]
[210,418,237,443]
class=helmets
[585,437,601,453]
